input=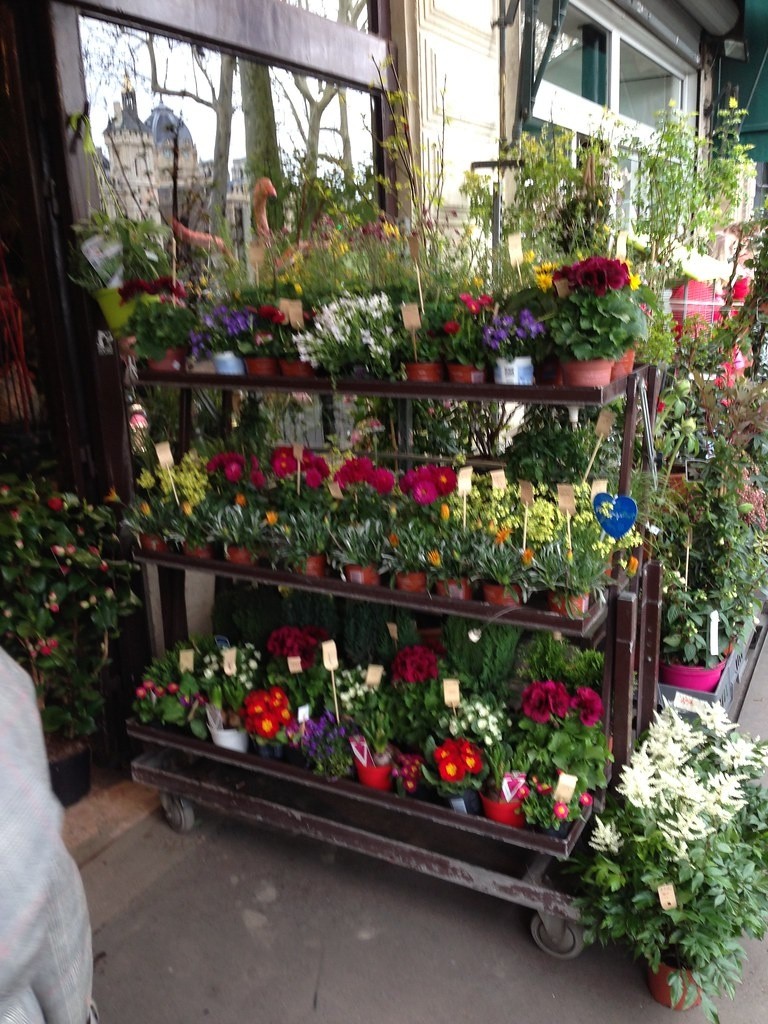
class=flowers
[133,623,616,834]
[570,693,768,1024]
[116,257,649,362]
[134,448,643,590]
[0,489,134,737]
[646,521,708,665]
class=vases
[482,585,523,607]
[650,959,704,1010]
[396,572,428,593]
[436,577,473,600]
[344,564,378,587]
[140,349,638,386]
[479,789,526,827]
[207,725,248,754]
[183,538,214,559]
[45,733,91,805]
[355,757,392,792]
[294,556,324,577]
[225,546,255,566]
[659,652,726,694]
[140,534,169,552]
[551,592,589,616]
[445,791,477,815]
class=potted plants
[70,208,173,333]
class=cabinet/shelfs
[100,337,664,929]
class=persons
[0,645,98,1024]
